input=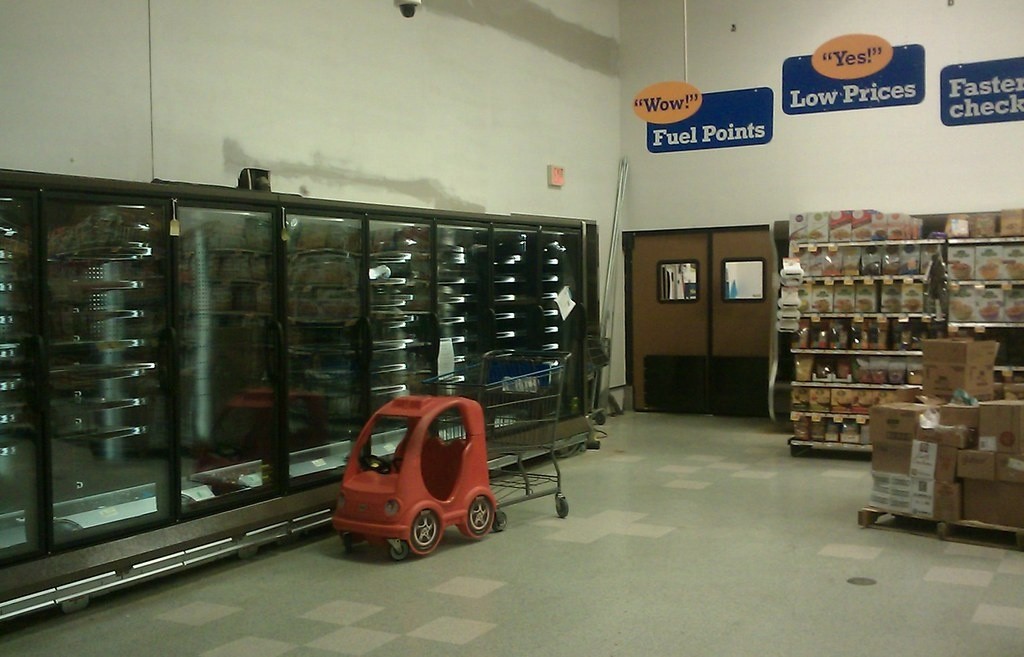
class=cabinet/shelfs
[778,206,1023,456]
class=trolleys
[420,347,573,531]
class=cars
[330,394,500,561]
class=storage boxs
[868,338,1024,530]
[789,318,879,445]
[788,209,1024,328]
